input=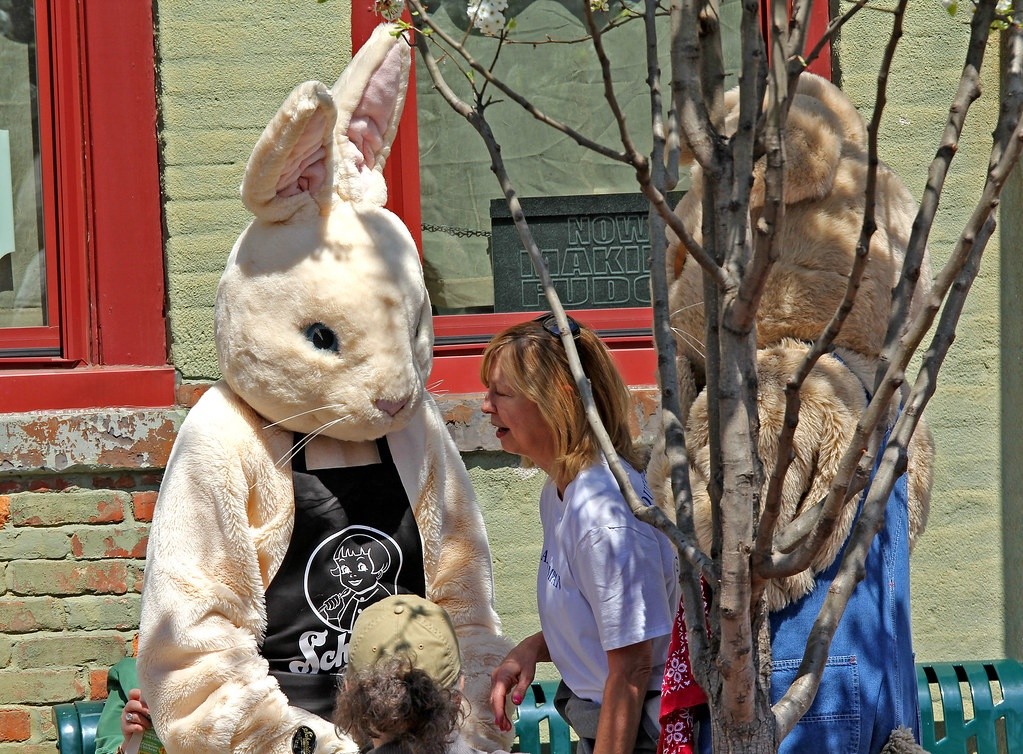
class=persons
[94,657,153,754]
[333,593,471,754]
[480,312,681,754]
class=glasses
[532,311,582,356]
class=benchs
[51,658,1023,754]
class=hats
[346,593,460,693]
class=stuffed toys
[665,70,935,754]
[136,23,518,754]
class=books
[124,714,167,754]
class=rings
[126,713,133,722]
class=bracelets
[117,747,124,754]
